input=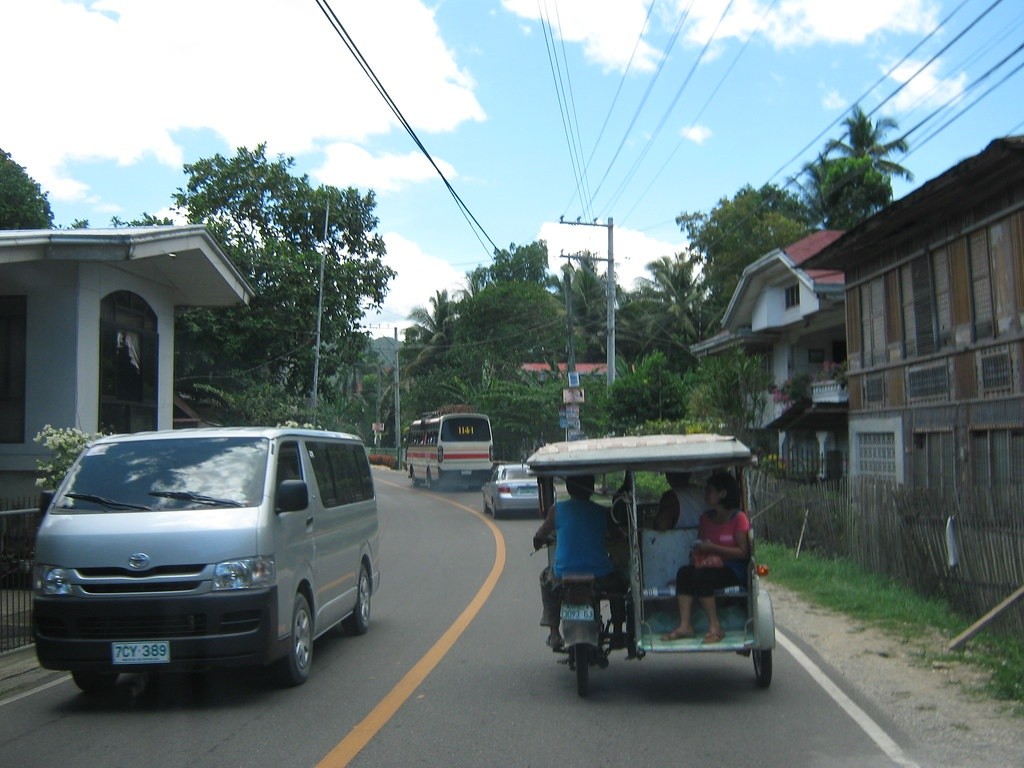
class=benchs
[643,583,749,603]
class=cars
[482,465,556,520]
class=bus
[403,414,494,490]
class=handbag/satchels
[690,540,724,568]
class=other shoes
[546,635,562,648]
[610,638,624,649]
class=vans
[31,427,378,693]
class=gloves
[533,536,542,550]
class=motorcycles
[527,434,776,698]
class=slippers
[662,629,696,641]
[704,630,725,643]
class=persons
[534,475,626,650]
[653,472,716,530]
[660,472,750,643]
